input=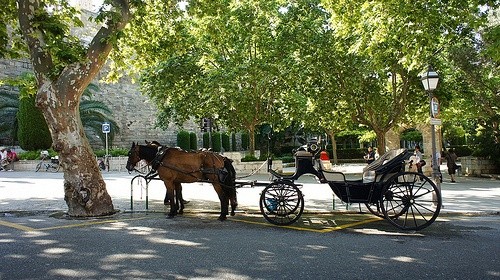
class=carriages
[125,139,442,231]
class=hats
[7,149,11,151]
[2,147,5,150]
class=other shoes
[183,200,190,204]
[8,169,14,172]
[450,180,455,183]
[164,201,170,205]
[1,169,6,171]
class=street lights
[421,70,445,210]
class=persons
[320,149,329,160]
[0,148,19,171]
[441,149,457,183]
[363,146,379,165]
[97,158,105,171]
[414,145,424,182]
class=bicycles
[35,151,61,173]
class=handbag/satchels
[419,160,426,166]
[454,164,458,169]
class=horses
[126,140,239,221]
